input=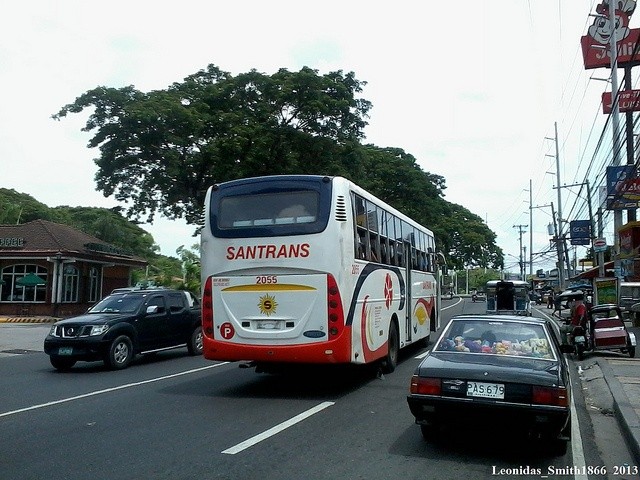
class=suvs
[44,287,203,369]
[486,279,532,316]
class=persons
[547,294,554,309]
[471,288,478,299]
[560,295,587,346]
[552,293,565,318]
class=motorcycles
[534,295,541,305]
[559,317,588,361]
[473,296,476,302]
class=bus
[201,175,448,373]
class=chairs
[233,219,252,227]
[254,218,274,226]
[276,217,295,225]
[357,233,396,267]
[297,216,316,224]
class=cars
[475,291,485,301]
[407,315,575,454]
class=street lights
[52,250,63,317]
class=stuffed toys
[438,334,552,360]
